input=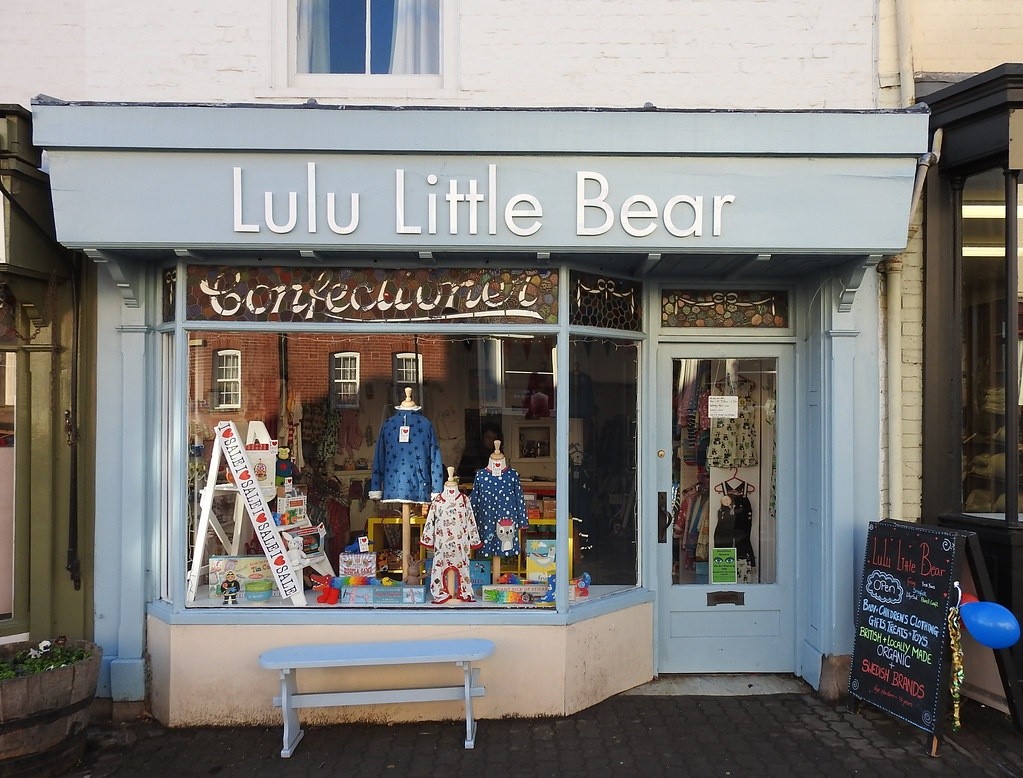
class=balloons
[958,587,980,631]
[960,601,1020,648]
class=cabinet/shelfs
[368,517,574,586]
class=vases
[0,640,105,778]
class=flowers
[0,634,95,678]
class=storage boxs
[276,495,306,524]
[338,536,574,604]
[282,526,323,558]
[208,554,281,600]
[523,492,556,519]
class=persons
[456,422,504,477]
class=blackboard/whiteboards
[848,518,968,735]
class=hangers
[714,467,757,496]
[694,473,709,490]
[714,362,757,391]
[569,441,592,462]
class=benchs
[258,638,497,759]
